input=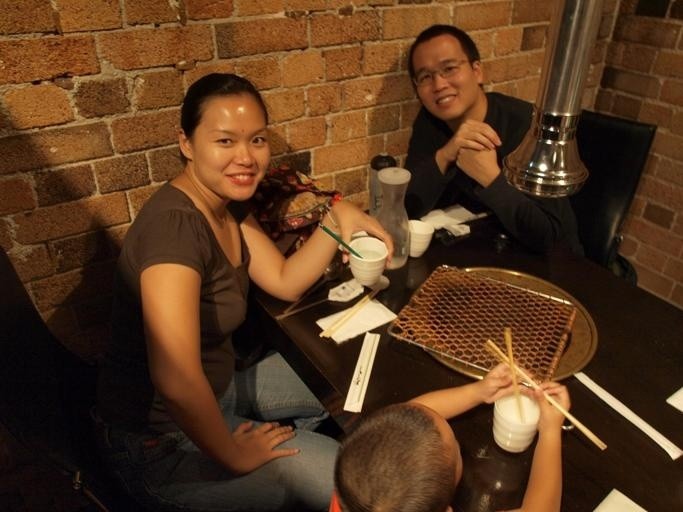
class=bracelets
[327,209,339,226]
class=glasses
[412,60,471,86]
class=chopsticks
[318,288,381,340]
[482,327,609,452]
[274,278,329,323]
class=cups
[347,236,389,287]
[491,394,541,455]
[408,220,436,259]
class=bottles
[370,151,398,219]
[367,166,413,272]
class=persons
[401,23,587,257]
[330,360,573,512]
[99,72,395,511]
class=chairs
[575,109,657,285]
[0,247,135,512]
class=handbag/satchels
[247,167,343,257]
